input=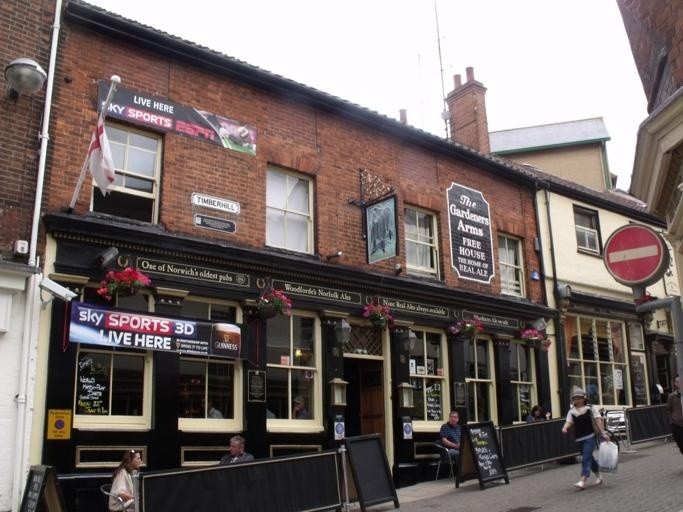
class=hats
[571,388,587,402]
[293,395,304,404]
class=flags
[86,113,116,197]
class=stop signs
[602,223,665,284]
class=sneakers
[574,481,585,489]
[594,475,602,484]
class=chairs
[433,439,456,481]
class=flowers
[258,288,293,319]
[362,303,393,331]
[96,267,151,301]
[449,317,485,339]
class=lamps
[3,56,48,100]
[399,325,416,365]
[332,318,351,372]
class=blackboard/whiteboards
[343,433,396,506]
[20,465,48,512]
[465,421,506,481]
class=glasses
[130,449,135,454]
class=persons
[665,375,682,456]
[588,384,598,398]
[293,397,308,419]
[201,398,223,418]
[528,406,545,422]
[267,410,275,419]
[224,436,253,463]
[561,391,608,489]
[439,411,461,472]
[108,450,142,512]
